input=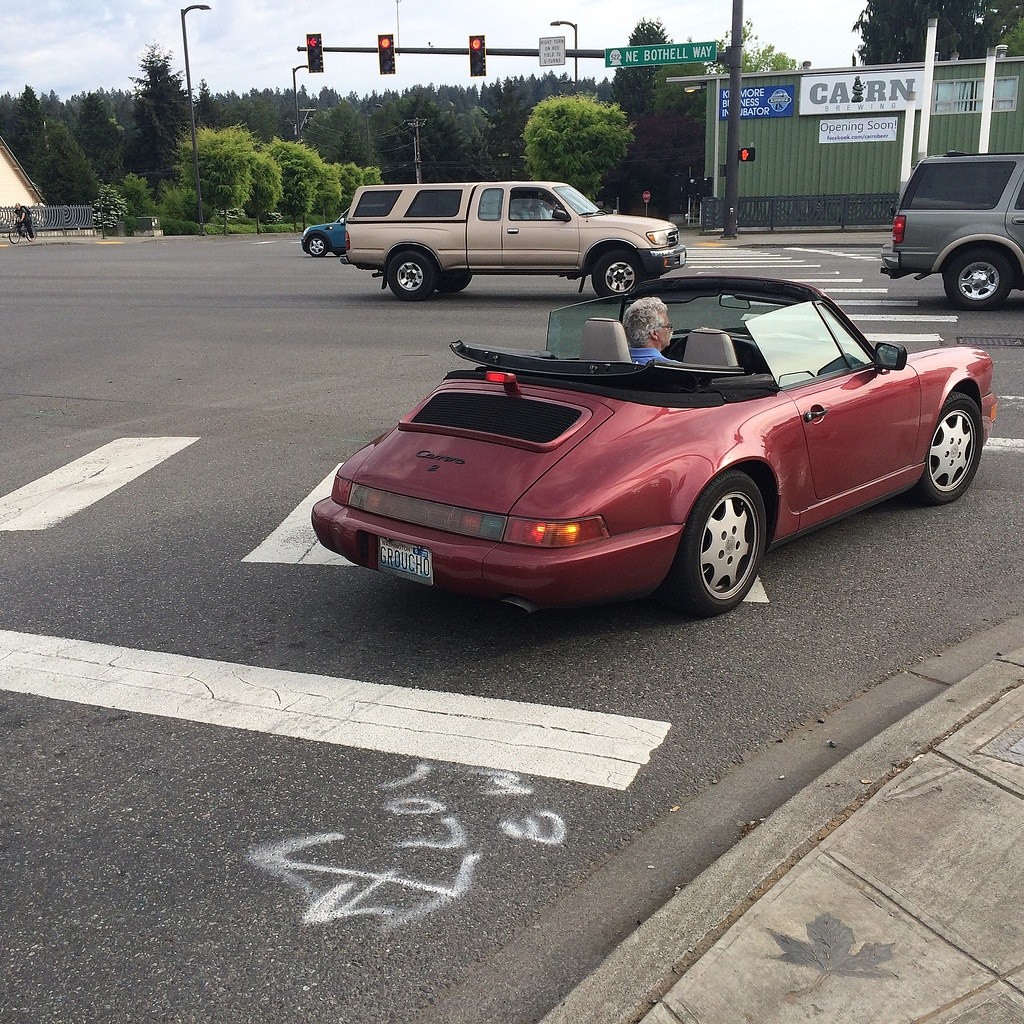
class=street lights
[550,20,578,94]
[365,102,382,167]
[180,3,211,239]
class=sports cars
[309,275,998,619]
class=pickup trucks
[340,181,686,302]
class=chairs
[683,327,738,368]
[578,316,633,363]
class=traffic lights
[738,146,755,160]
[378,33,396,75]
[468,35,486,77]
[306,34,324,74]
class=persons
[13,203,36,241]
[622,296,682,364]
[535,199,557,219]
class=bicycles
[8,217,38,244]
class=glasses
[658,321,674,328]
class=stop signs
[642,191,650,200]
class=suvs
[300,204,388,258]
[881,150,1024,311]
